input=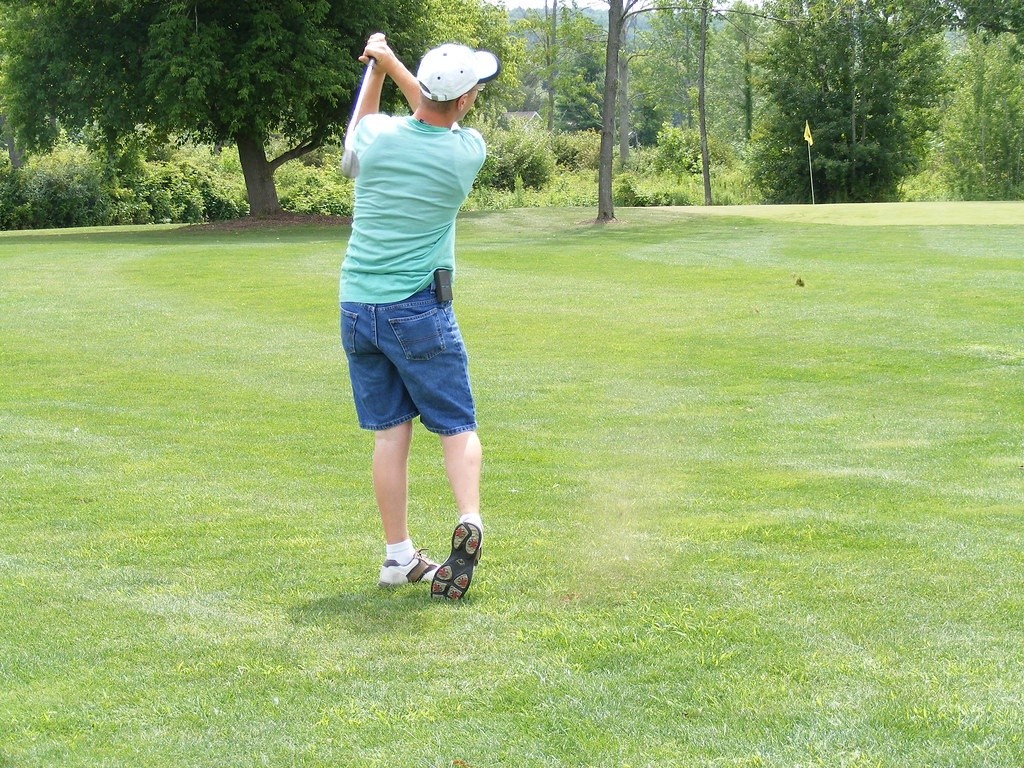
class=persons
[340,34,500,602]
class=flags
[804,120,813,146]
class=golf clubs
[340,56,376,178]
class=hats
[417,43,501,104]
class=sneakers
[431,517,484,603]
[377,548,441,585]
